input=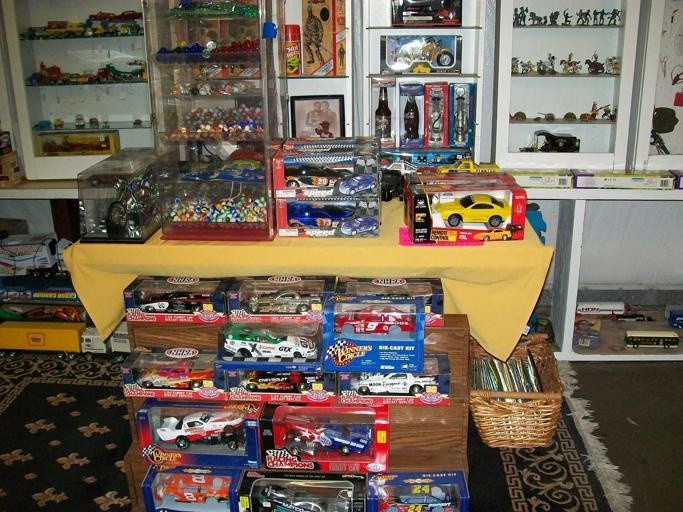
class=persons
[318,101,336,137]
[511,6,622,122]
[314,120,333,138]
[305,101,323,137]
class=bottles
[403,97,418,141]
[374,87,390,146]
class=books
[472,350,543,403]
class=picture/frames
[291,94,345,138]
[633,0,683,172]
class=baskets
[470,335,562,446]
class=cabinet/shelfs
[361,0,486,165]
[2,0,153,181]
[144,0,278,240]
[493,2,638,174]
[122,315,471,512]
[555,189,683,360]
